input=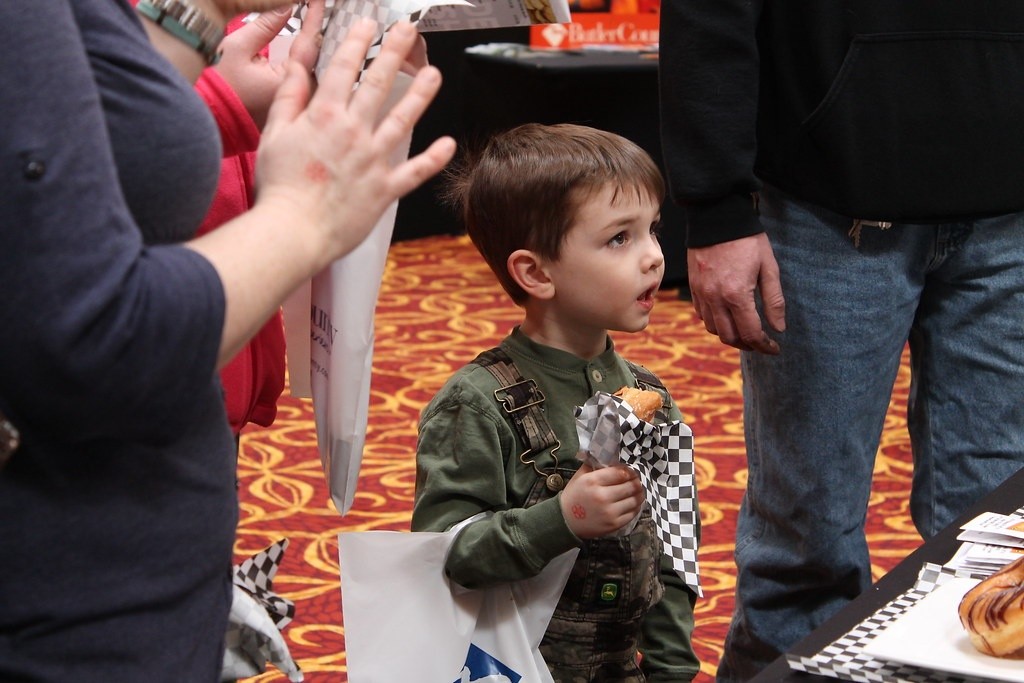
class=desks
[744,466,1024,683]
[468,43,686,294]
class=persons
[412,123,700,683]
[0,0,456,683]
[654,1,1023,683]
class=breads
[612,384,662,426]
[958,554,1024,657]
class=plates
[862,577,1024,682]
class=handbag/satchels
[337,510,580,683]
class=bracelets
[136,0,216,71]
[149,0,225,50]
